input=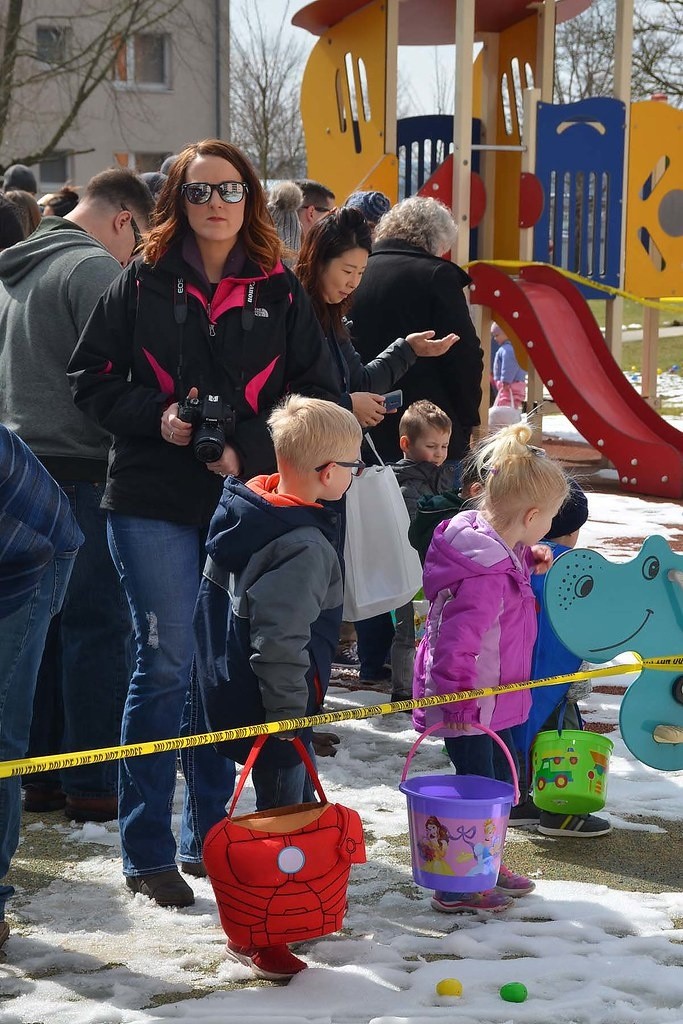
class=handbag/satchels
[488,388,519,430]
[200,735,368,951]
[343,434,423,626]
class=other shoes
[390,694,415,713]
[334,649,360,664]
[125,866,197,909]
[65,794,118,821]
[358,667,395,686]
[0,920,10,949]
[179,857,207,879]
[23,787,69,812]
[0,885,16,902]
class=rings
[220,472,226,477]
[170,432,173,439]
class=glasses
[120,202,147,258]
[314,458,366,479]
[180,179,252,205]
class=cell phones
[376,390,402,413]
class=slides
[466,259,683,499]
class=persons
[263,179,484,713]
[192,393,366,979]
[141,155,178,196]
[294,209,461,759]
[0,426,85,950]
[0,164,80,250]
[65,140,341,908]
[491,322,525,408]
[507,477,612,836]
[0,168,156,823]
[413,424,569,912]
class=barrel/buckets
[390,600,430,647]
[399,720,520,893]
[532,701,614,814]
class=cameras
[177,395,238,463]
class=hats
[343,190,391,224]
[539,472,589,539]
[4,163,38,195]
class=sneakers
[495,865,538,897]
[537,810,612,837]
[507,794,542,828]
[431,889,516,915]
[224,935,310,982]
